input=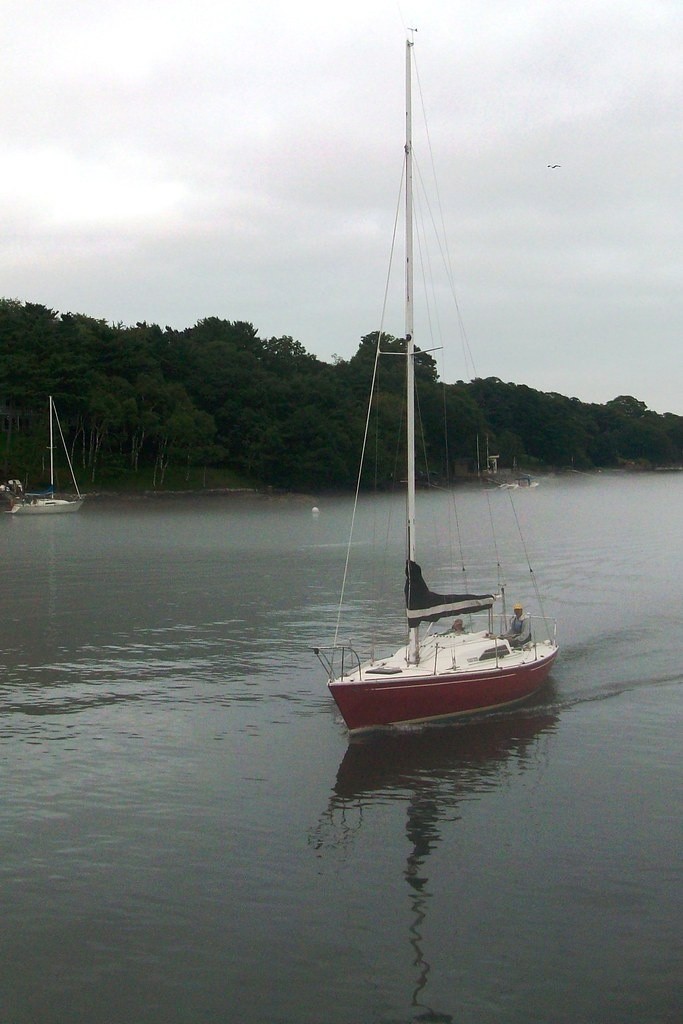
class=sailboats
[5,397,86,514]
[313,26,562,734]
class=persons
[502,604,531,648]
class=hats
[513,604,522,609]
